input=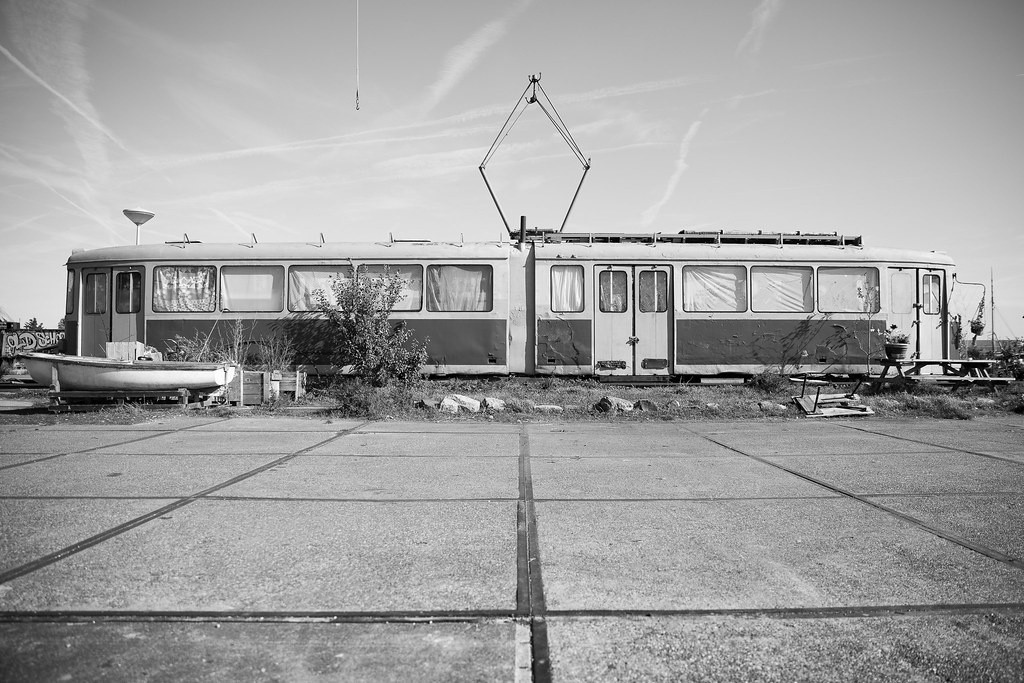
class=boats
[18,348,241,392]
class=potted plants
[969,320,986,336]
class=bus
[62,73,986,380]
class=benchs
[869,375,1017,384]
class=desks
[876,359,998,396]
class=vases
[883,344,908,360]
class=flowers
[869,323,910,344]
